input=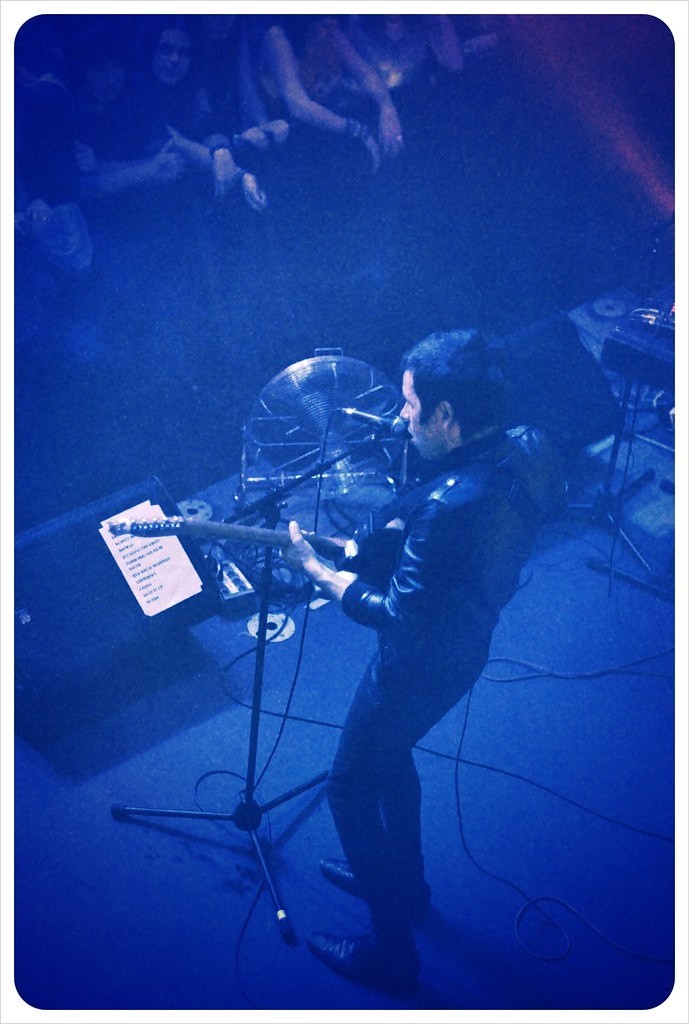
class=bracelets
[344,117,355,140]
[236,170,247,188]
[210,144,228,159]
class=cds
[247,612,296,643]
[594,300,627,317]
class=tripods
[566,228,664,579]
[111,431,383,946]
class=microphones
[337,407,405,433]
[653,210,675,235]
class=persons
[278,331,533,999]
[15,14,509,266]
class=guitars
[111,519,409,579]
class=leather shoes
[307,932,421,998]
[320,857,428,921]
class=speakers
[14,474,215,696]
[479,310,624,452]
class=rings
[43,217,46,220]
